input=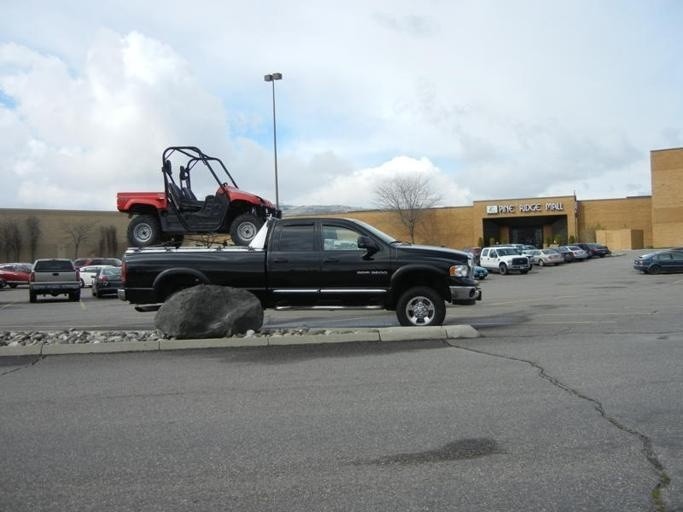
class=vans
[72,257,123,272]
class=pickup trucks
[29,258,81,303]
[118,216,482,329]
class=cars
[470,243,609,280]
[90,267,122,298]
[117,145,282,248]
[1,262,33,288]
[80,264,117,288]
[632,250,682,275]
